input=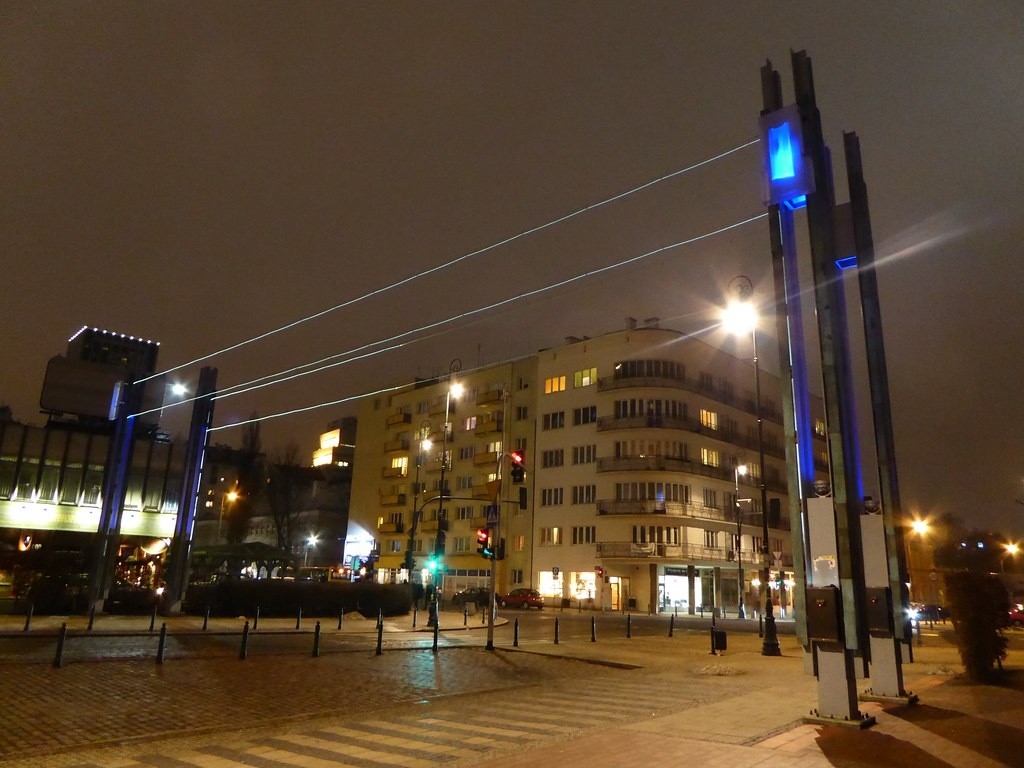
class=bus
[276,566,353,583]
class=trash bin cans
[714,630,727,651]
[463,601,475,616]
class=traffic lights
[593,565,605,579]
[510,450,528,486]
[475,527,494,562]
[429,559,438,572]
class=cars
[455,587,499,609]
[502,589,545,611]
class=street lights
[735,461,749,621]
[217,493,239,538]
[428,383,465,627]
[407,420,432,583]
[728,275,781,655]
